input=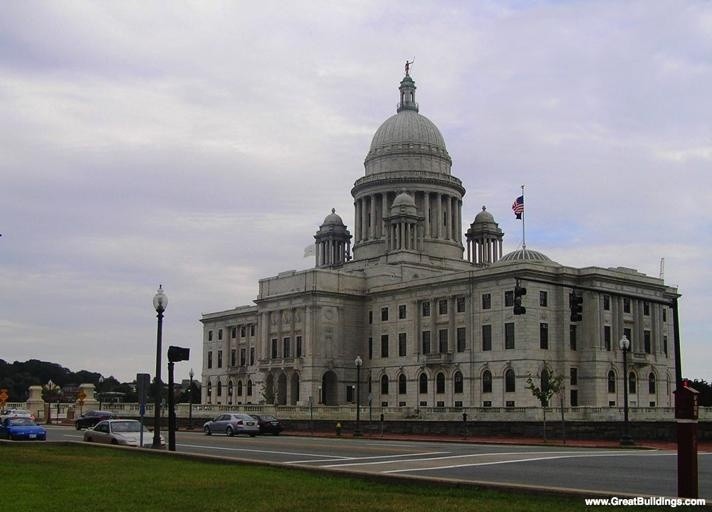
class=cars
[82,416,167,450]
[245,412,283,436]
[201,411,261,439]
[73,409,116,431]
[0,407,35,427]
[0,415,49,442]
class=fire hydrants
[336,423,343,436]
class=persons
[404,60,414,73]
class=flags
[512,195,524,220]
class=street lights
[352,351,364,437]
[151,282,169,449]
[318,385,323,405]
[97,374,104,410]
[186,367,194,431]
[46,378,54,425]
[55,383,60,424]
[351,384,356,404]
[619,332,633,447]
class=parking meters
[380,414,385,437]
[461,413,468,440]
